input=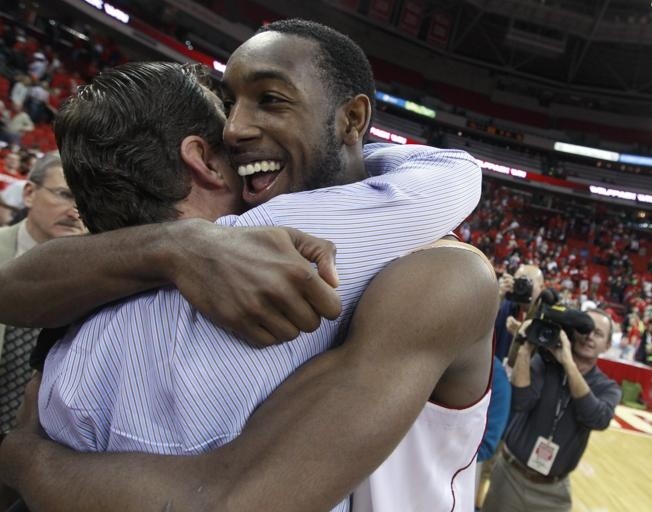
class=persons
[0,153,89,433]
[39,59,483,512]
[0,0,125,228]
[366,111,652,511]
[0,17,501,512]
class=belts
[502,445,567,483]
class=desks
[596,355,652,411]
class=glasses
[38,182,73,200]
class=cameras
[504,277,534,304]
